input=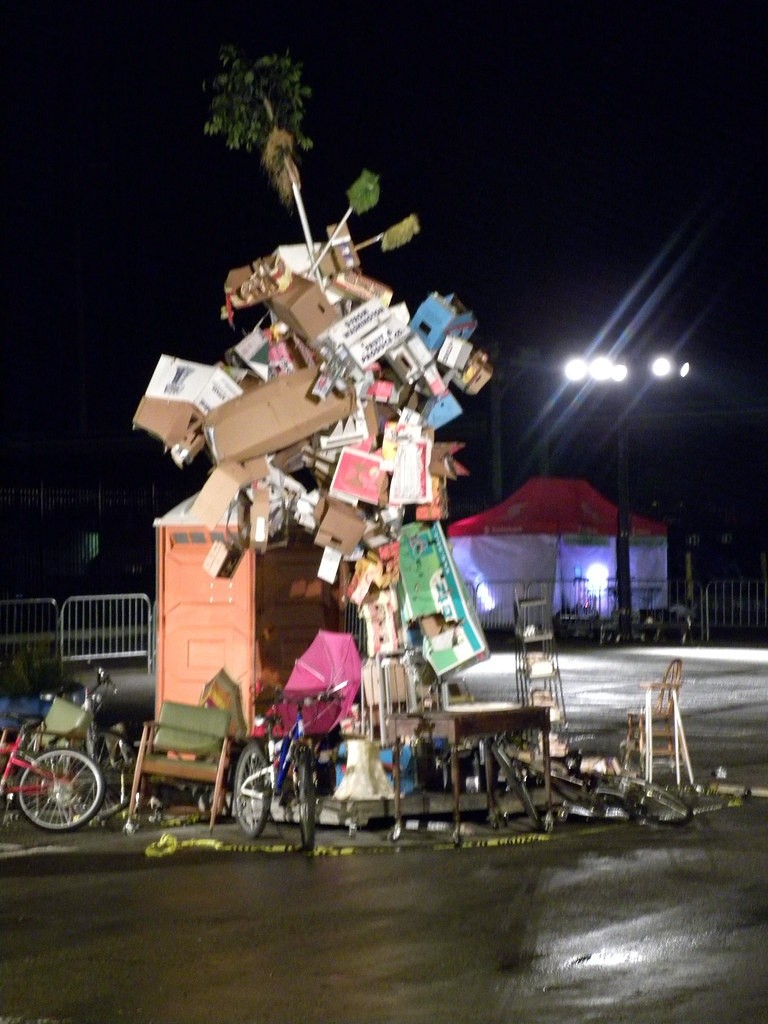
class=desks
[383,703,554,845]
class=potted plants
[0,632,84,728]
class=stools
[640,682,694,786]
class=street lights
[564,356,690,647]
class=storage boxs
[134,221,491,792]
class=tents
[446,476,668,624]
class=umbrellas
[280,629,362,735]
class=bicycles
[230,678,351,850]
[0,665,142,835]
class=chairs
[124,701,233,835]
[622,658,683,774]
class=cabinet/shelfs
[509,575,568,719]
